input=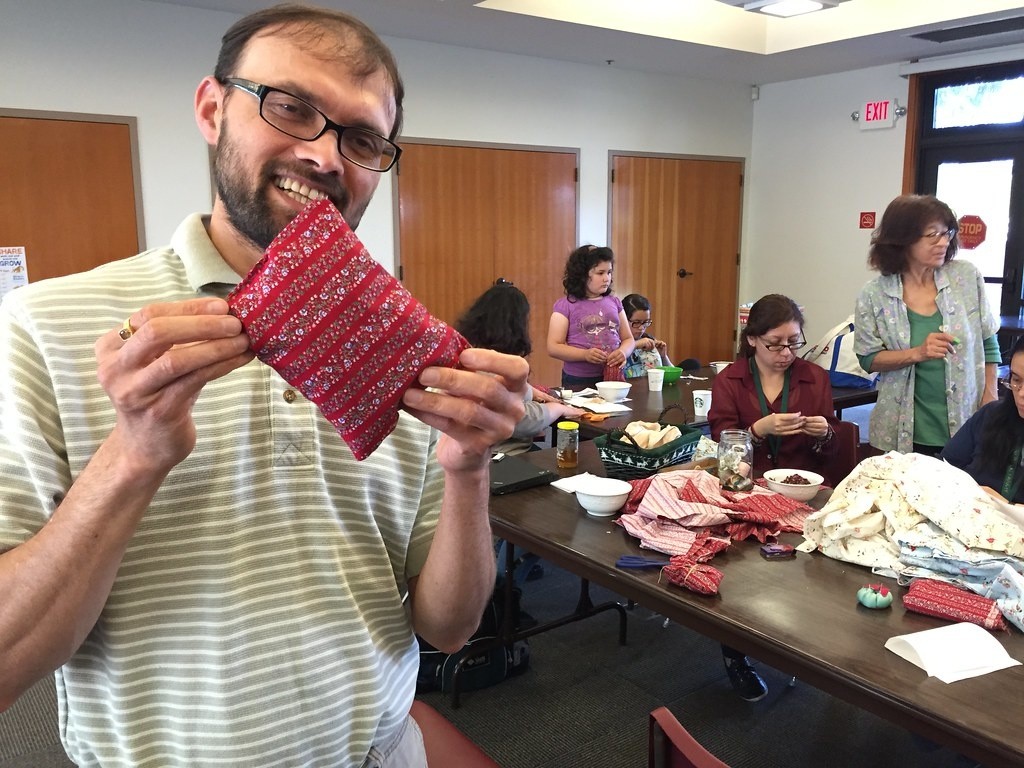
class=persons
[621,294,701,378]
[852,194,1002,455]
[454,277,587,583]
[706,294,854,703]
[939,333,1024,507]
[547,245,635,387]
[0,0,530,768]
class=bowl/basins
[596,381,632,400]
[654,366,683,382]
[710,361,734,374]
[763,469,824,505]
[575,478,633,516]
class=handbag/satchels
[415,576,525,693]
[801,312,883,390]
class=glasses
[758,329,807,351]
[921,229,957,245]
[220,77,403,172]
[628,319,652,328]
[1000,373,1024,391]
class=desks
[489,363,1024,768]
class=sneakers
[723,655,767,703]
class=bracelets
[816,427,832,445]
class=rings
[118,318,134,342]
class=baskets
[594,403,703,481]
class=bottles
[717,430,754,491]
[557,421,579,468]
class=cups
[692,390,712,417]
[647,369,664,391]
[716,364,728,373]
